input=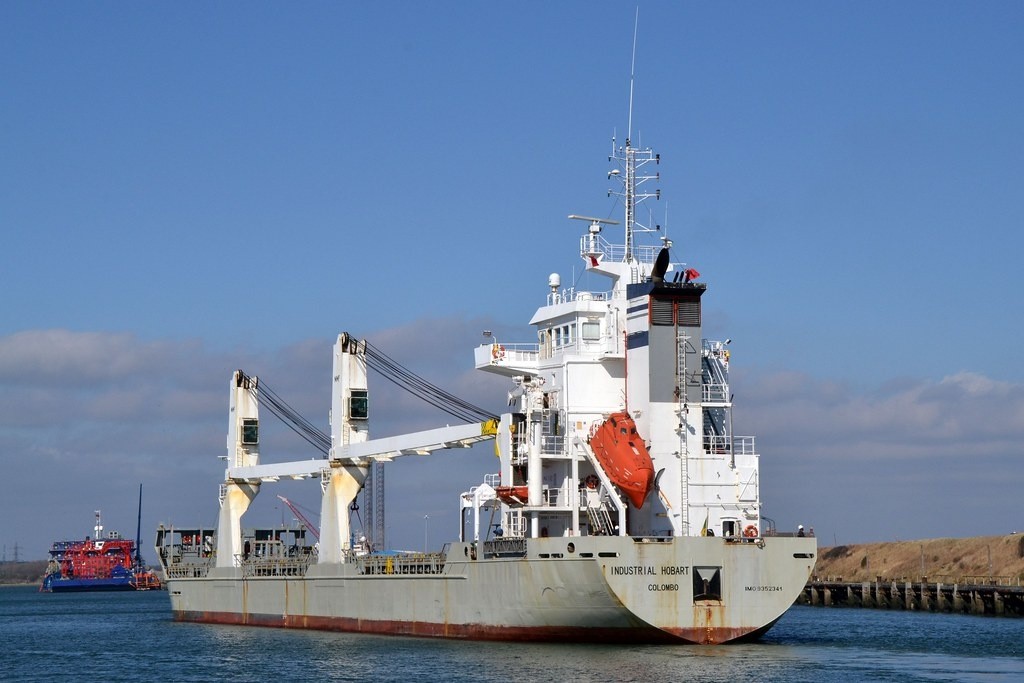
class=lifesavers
[743,525,759,537]
[492,345,506,358]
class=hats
[798,525,803,530]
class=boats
[155,6,817,645]
[38,483,161,592]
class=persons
[797,525,805,537]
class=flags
[701,516,708,537]
